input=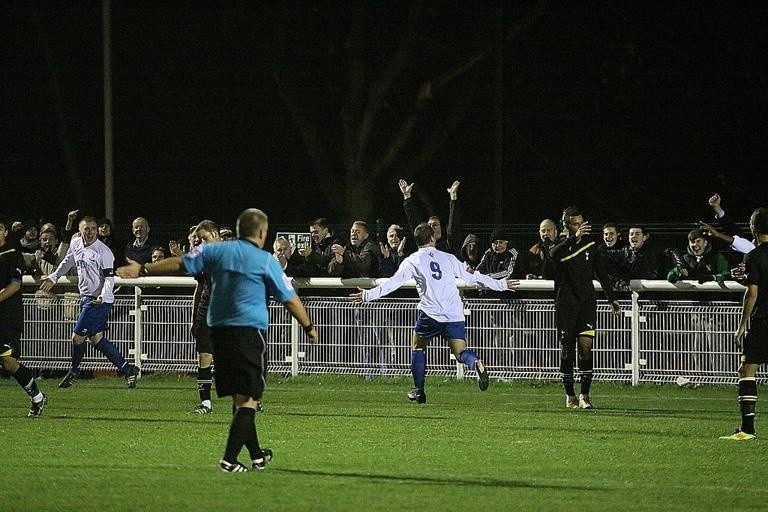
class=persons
[539,204,625,410]
[97,214,216,379]
[273,220,415,380]
[186,218,266,417]
[717,206,768,442]
[398,179,568,382]
[0,219,48,419]
[347,224,521,405]
[598,194,758,386]
[114,207,320,474]
[39,219,139,387]
[21,208,96,379]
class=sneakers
[401,386,429,405]
[717,426,759,442]
[216,455,251,475]
[123,362,141,389]
[563,387,595,410]
[473,356,491,394]
[25,394,49,420]
[57,369,82,389]
[248,446,275,471]
[185,404,215,417]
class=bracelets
[304,323,315,333]
[140,264,148,275]
